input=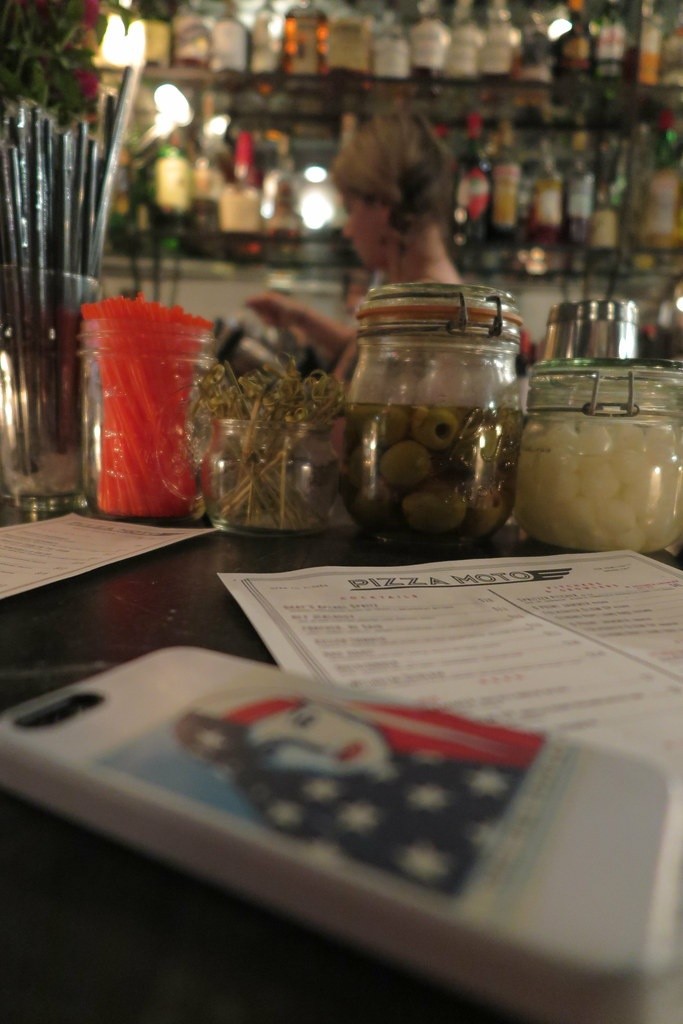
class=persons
[245,112,463,381]
[183,692,548,900]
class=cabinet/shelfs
[98,0,683,284]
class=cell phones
[0,646,683,1024]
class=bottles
[560,0,595,78]
[155,147,188,210]
[608,136,631,243]
[409,0,449,79]
[632,0,663,84]
[642,109,683,252]
[327,0,371,77]
[174,0,208,70]
[284,0,327,77]
[596,0,627,78]
[482,0,520,73]
[563,133,595,245]
[531,139,563,244]
[595,192,617,249]
[371,0,410,80]
[250,0,284,74]
[494,120,519,233]
[443,0,483,78]
[210,0,250,71]
[456,109,490,240]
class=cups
[210,419,344,534]
[80,317,220,519]
[0,266,99,509]
